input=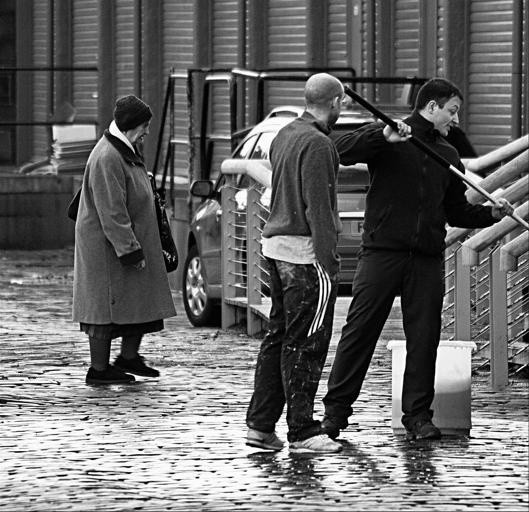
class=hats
[113,94,153,134]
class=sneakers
[287,432,344,455]
[319,418,341,441]
[112,355,161,380]
[245,428,286,452]
[83,364,137,387]
[402,419,441,442]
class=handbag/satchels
[66,175,181,273]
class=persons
[68,91,178,386]
[319,76,516,446]
[241,73,345,457]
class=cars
[182,105,487,329]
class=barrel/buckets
[386,339,477,436]
